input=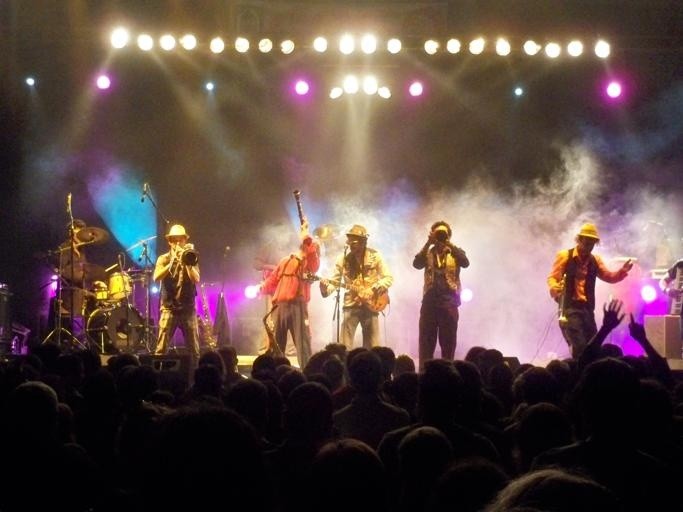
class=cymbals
[62,263,105,282]
[76,227,109,246]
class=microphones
[66,195,70,217]
[344,245,348,250]
[141,184,146,202]
[139,247,146,261]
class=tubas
[313,224,347,271]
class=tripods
[117,275,176,355]
[39,238,88,352]
[51,207,110,356]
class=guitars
[308,272,389,313]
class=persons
[318,220,396,356]
[411,218,470,374]
[244,217,322,373]
[0,297,682,511]
[150,222,201,370]
[543,218,634,363]
[41,218,99,353]
[658,255,683,317]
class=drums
[86,302,144,354]
[60,288,86,317]
[109,273,132,300]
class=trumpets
[434,230,447,247]
[557,274,568,323]
[174,247,199,265]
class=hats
[164,224,191,238]
[345,224,369,241]
[576,223,602,241]
